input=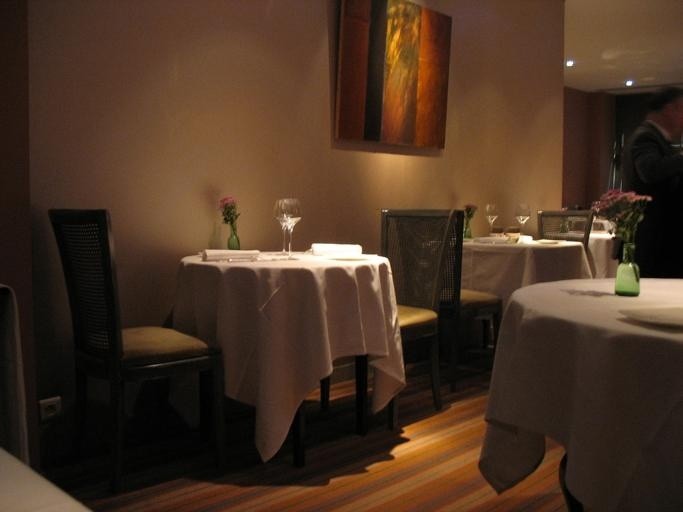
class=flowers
[590,188,653,284]
[217,196,240,247]
[463,202,479,235]
[558,207,568,232]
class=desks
[508,276,682,511]
[180,251,389,468]
[544,231,619,278]
[421,237,583,349]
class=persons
[620,86,682,277]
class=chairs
[536,209,593,254]
[45,207,228,497]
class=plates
[479,236,510,242]
[618,305,682,329]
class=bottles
[227,223,240,250]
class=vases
[227,223,240,250]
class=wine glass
[272,199,303,259]
[515,204,531,235]
[485,203,497,235]
